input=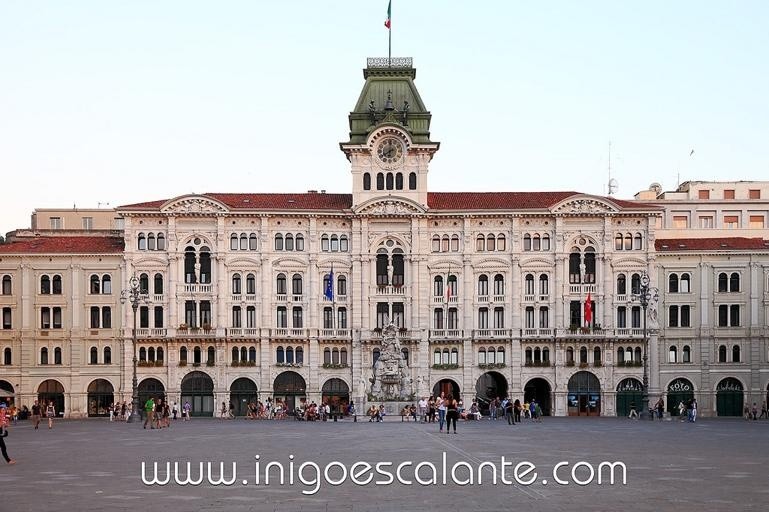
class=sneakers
[369,419,383,423]
[508,422,517,425]
[8,459,16,465]
[143,424,170,429]
[439,430,457,434]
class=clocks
[376,136,403,163]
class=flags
[442,263,451,307]
[326,261,334,302]
[585,284,592,323]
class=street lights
[118,271,153,413]
[630,270,660,412]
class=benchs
[351,413,421,422]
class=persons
[742,402,751,420]
[752,402,758,421]
[443,393,459,434]
[628,400,639,419]
[435,391,446,433]
[0,402,57,465]
[759,401,768,419]
[107,398,193,430]
[650,396,698,423]
